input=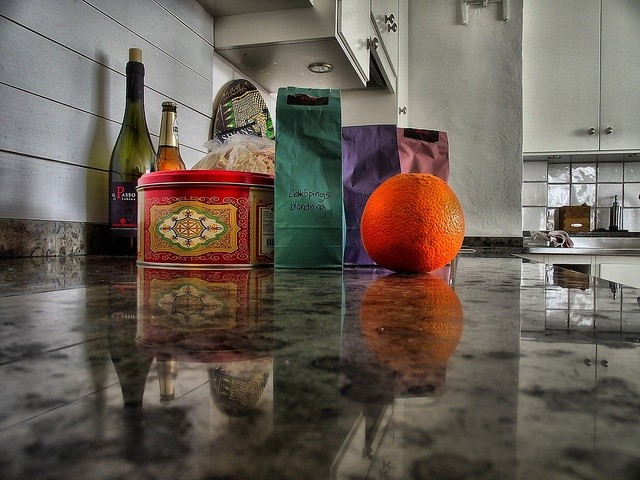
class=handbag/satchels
[274,86,343,269]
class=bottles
[609,281,620,300]
[609,195,623,230]
[107,48,158,258]
[157,355,177,408]
[156,102,186,172]
[104,261,156,446]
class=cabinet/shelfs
[398,0,408,125]
[521,1,639,156]
[214,1,369,93]
[371,0,398,93]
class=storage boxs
[560,205,591,232]
